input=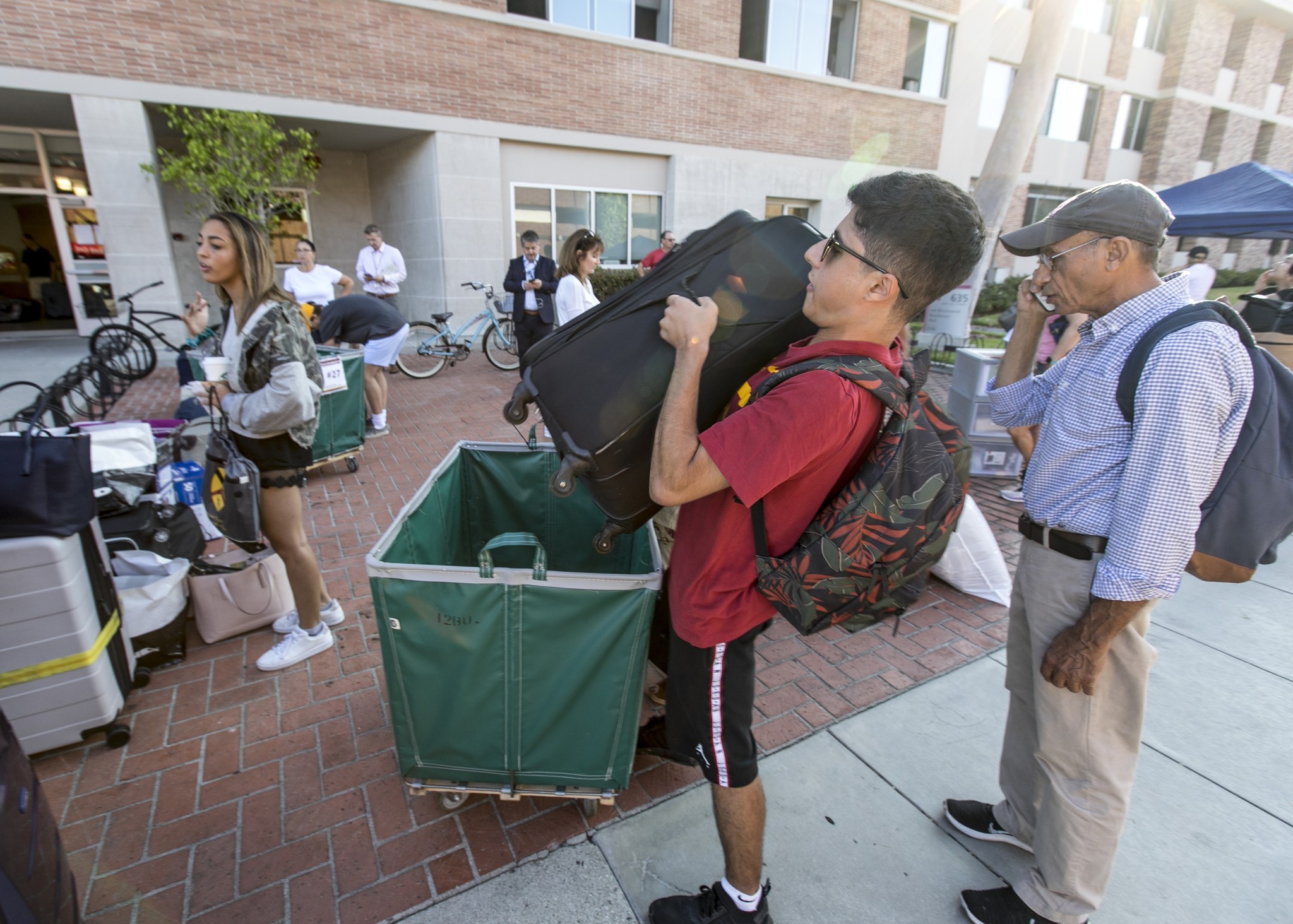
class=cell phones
[529,279,535,282]
[1034,286,1056,312]
[365,274,373,278]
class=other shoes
[1001,487,1026,503]
[365,416,389,439]
[637,714,699,768]
[648,677,669,706]
[649,876,773,924]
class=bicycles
[395,282,520,379]
[89,282,185,380]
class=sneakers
[254,623,335,671]
[946,799,1036,854]
[959,886,1089,924]
[274,598,344,634]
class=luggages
[0,516,151,750]
[503,208,829,554]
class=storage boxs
[949,347,1022,477]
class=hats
[299,300,318,333]
[999,179,1175,257]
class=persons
[503,231,559,380]
[19,233,55,321]
[636,230,676,278]
[1167,246,1209,276]
[637,171,989,923]
[1215,255,1293,307]
[1000,313,1090,502]
[944,180,1255,924]
[284,239,354,344]
[181,212,346,672]
[300,294,411,438]
[544,229,601,441]
[356,224,406,374]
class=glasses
[1042,236,1110,271]
[579,230,594,246]
[820,232,909,301]
[663,237,676,242]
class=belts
[1018,513,1107,561]
[525,309,539,315]
[367,292,395,298]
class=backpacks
[750,356,973,639]
[1114,298,1292,583]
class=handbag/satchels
[68,414,224,668]
[0,380,100,539]
[202,385,267,546]
[189,542,295,644]
[1048,317,1069,344]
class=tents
[1154,162,1293,238]
